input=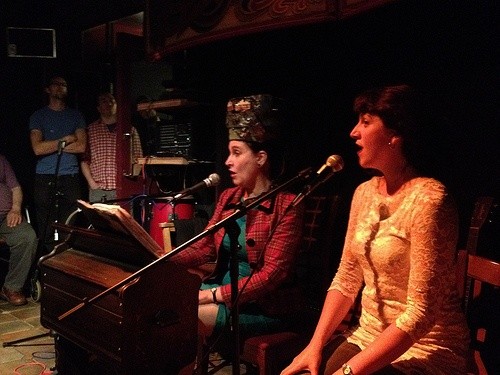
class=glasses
[47,80,68,88]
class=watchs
[210,287,217,303]
[342,364,352,375]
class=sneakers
[0,284,27,305]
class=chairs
[195,195,345,375]
[456,249,500,375]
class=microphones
[57,140,66,156]
[286,154,344,212]
[168,173,221,204]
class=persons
[279,81,467,375]
[160,97,306,375]
[0,155,40,307]
[83,91,144,204]
[30,75,87,277]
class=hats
[227,95,284,143]
